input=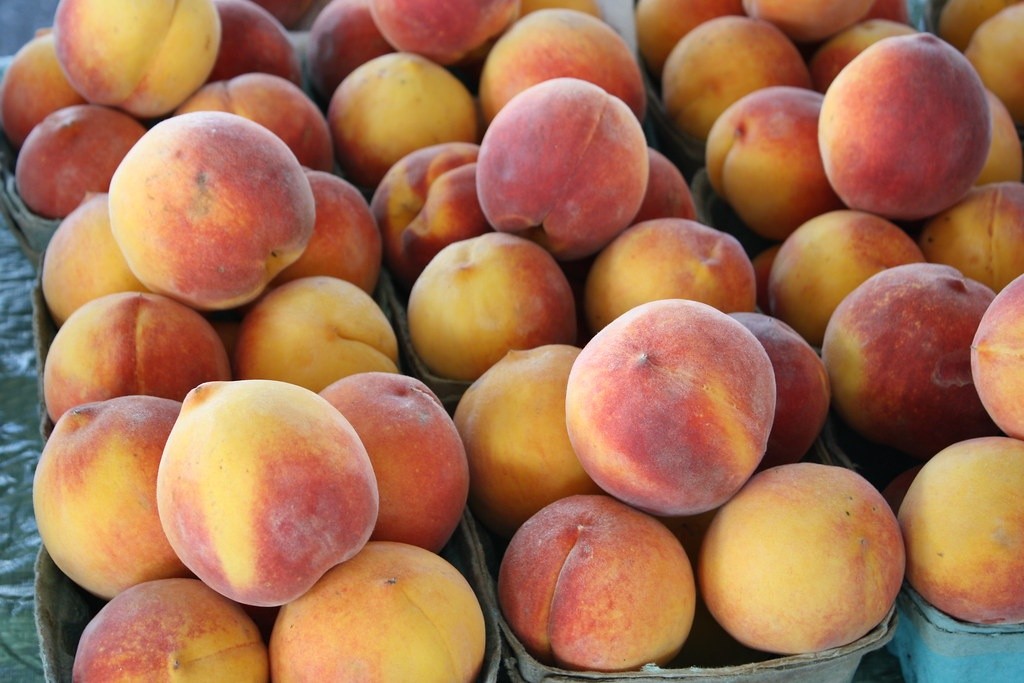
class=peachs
[2,0,1024,683]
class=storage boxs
[0,0,1024,683]
